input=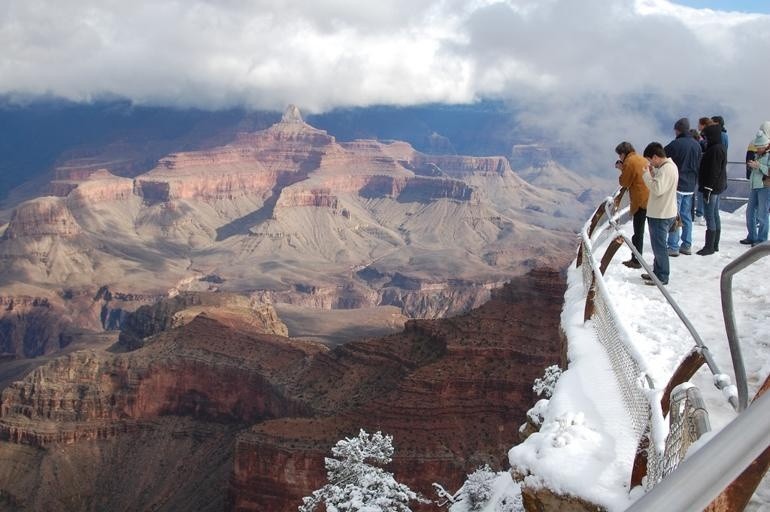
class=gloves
[703,187,713,204]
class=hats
[754,130,770,146]
[675,118,689,130]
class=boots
[697,229,720,255]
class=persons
[614,116,728,285]
[740,121,770,247]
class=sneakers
[623,260,642,268]
[695,215,706,225]
[667,248,679,256]
[679,245,692,255]
[642,272,655,279]
[740,239,756,244]
[646,279,665,285]
[752,240,763,247]
